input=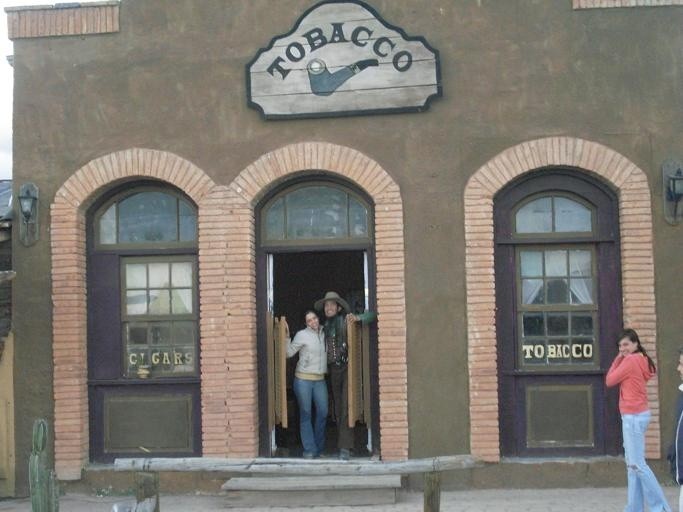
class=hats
[314,291,352,314]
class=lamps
[17,190,36,235]
[667,168,683,219]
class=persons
[285,310,330,458]
[665,348,683,511]
[604,328,672,511]
[313,291,375,460]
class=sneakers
[340,448,357,460]
[303,452,313,459]
